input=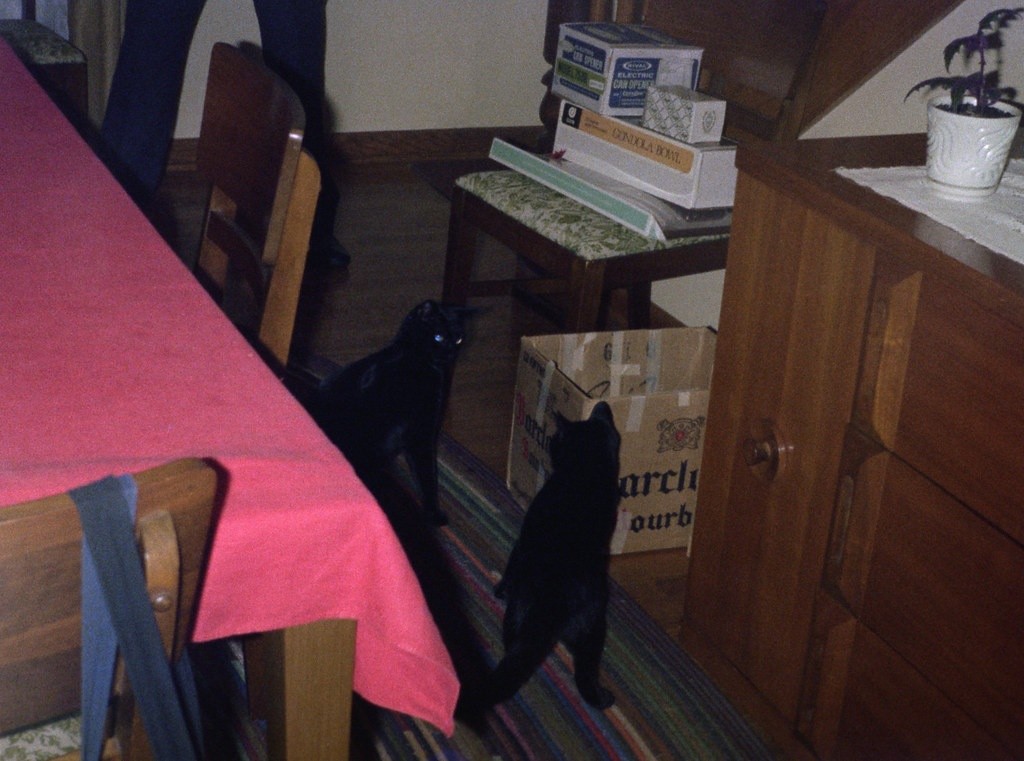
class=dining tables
[1,22,371,761]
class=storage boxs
[502,325,724,563]
[489,13,738,239]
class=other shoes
[306,189,352,270]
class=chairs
[444,0,837,383]
[198,39,326,372]
[1,451,225,761]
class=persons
[100,0,344,276]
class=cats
[252,296,475,526]
[462,401,622,716]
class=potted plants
[920,6,1024,201]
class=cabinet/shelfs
[672,124,1024,761]
